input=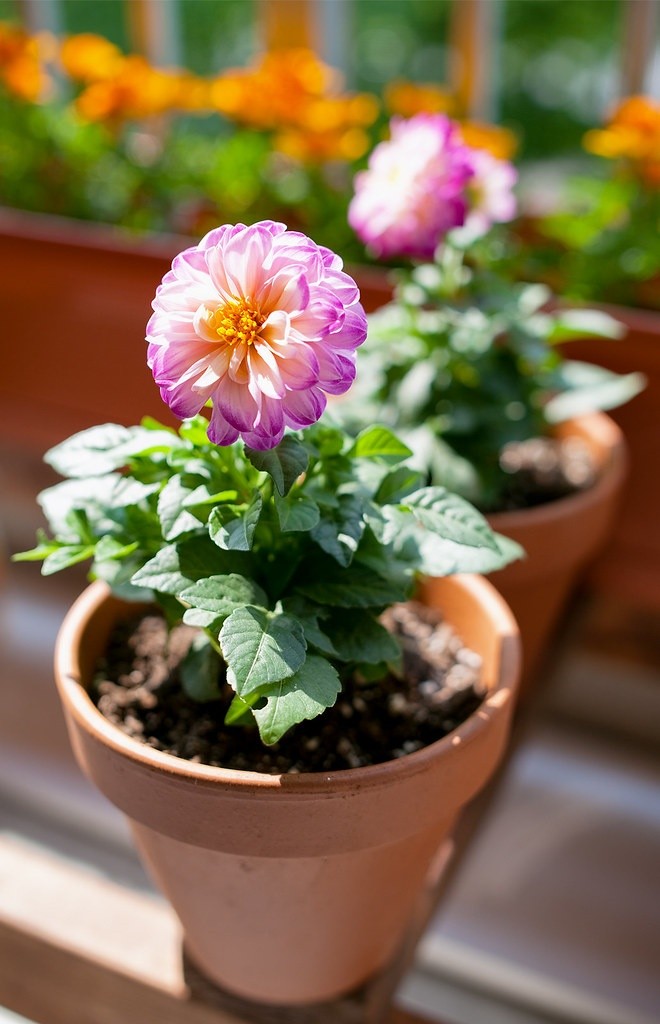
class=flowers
[0,215,531,748]
[0,20,660,318]
[319,110,651,514]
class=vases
[51,571,525,1007]
[448,398,629,686]
[0,200,660,671]
